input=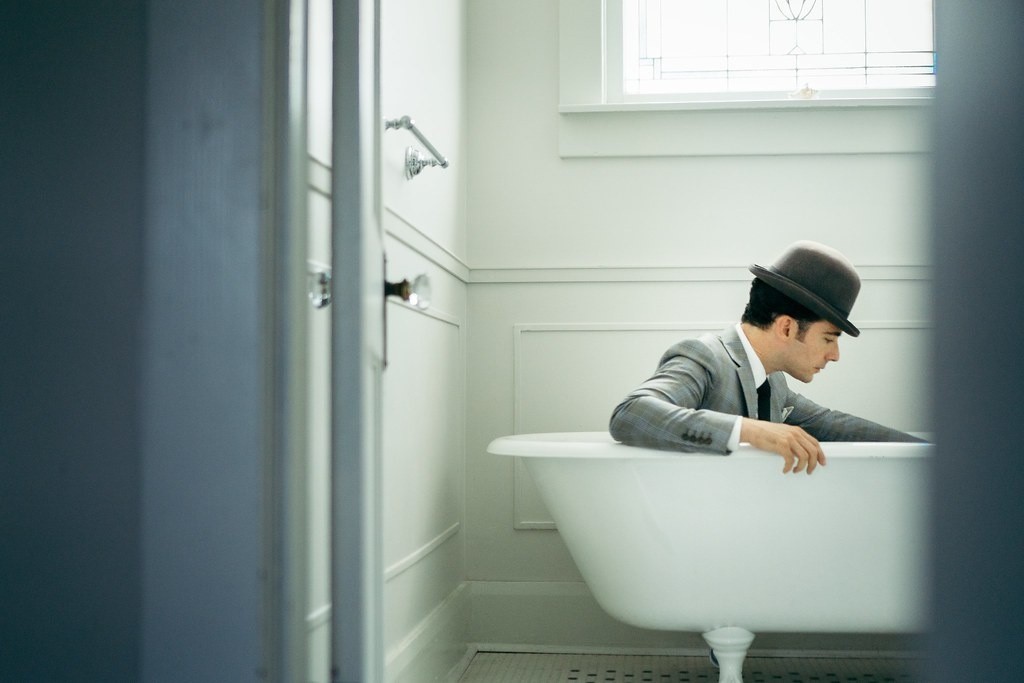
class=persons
[609,242,930,475]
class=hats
[747,240,862,338]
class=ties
[756,377,771,422]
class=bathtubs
[486,430,927,683]
